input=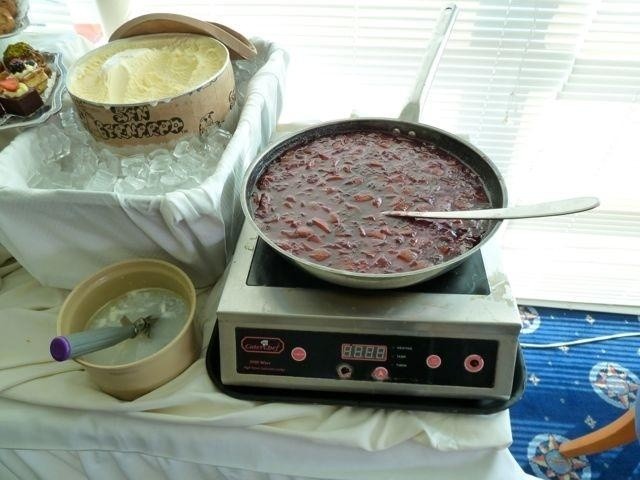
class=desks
[0,258,516,479]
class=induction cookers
[215,128,522,402]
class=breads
[0,0,16,35]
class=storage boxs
[1,30,293,292]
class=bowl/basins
[56,257,208,402]
[239,117,511,290]
[64,35,239,153]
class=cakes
[0,42,53,119]
